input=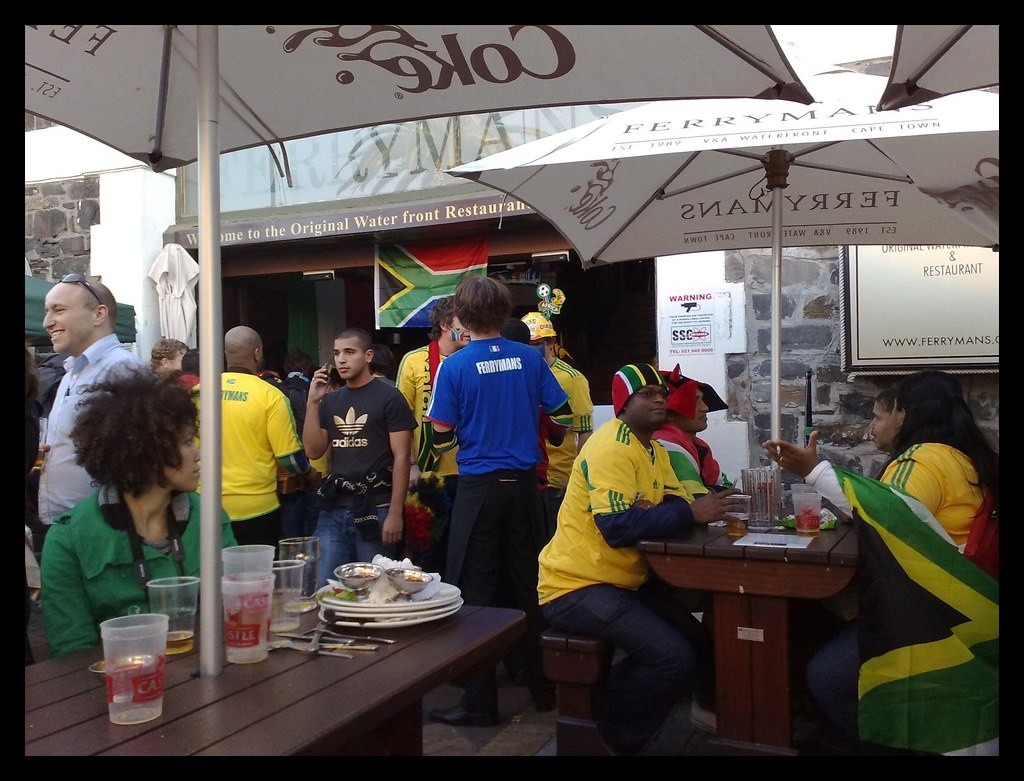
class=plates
[317,581,464,629]
[88,653,156,680]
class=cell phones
[321,359,333,385]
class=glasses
[58,273,105,304]
[635,387,669,400]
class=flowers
[402,490,437,552]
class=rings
[779,457,783,461]
[647,505,651,509]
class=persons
[537,363,741,733]
[760,373,999,756]
[40,364,239,661]
[652,371,739,508]
[25,272,593,731]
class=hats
[611,363,668,417]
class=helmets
[521,311,557,341]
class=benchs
[539,625,615,757]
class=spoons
[268,639,354,659]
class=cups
[221,543,276,582]
[278,536,321,615]
[220,573,276,665]
[741,467,782,533]
[791,484,822,538]
[270,560,307,634]
[147,575,200,656]
[724,494,751,542]
[99,613,170,725]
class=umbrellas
[25,25,814,678]
[445,91,1000,513]
[875,25,1000,115]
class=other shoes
[427,705,498,726]
[595,715,633,754]
[686,700,716,736]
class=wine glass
[333,561,384,599]
[385,568,434,604]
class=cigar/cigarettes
[776,444,781,454]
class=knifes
[306,621,327,654]
[274,632,356,645]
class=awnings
[163,181,538,254]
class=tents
[25,274,137,345]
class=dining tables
[641,482,861,754]
[24,596,526,756]
